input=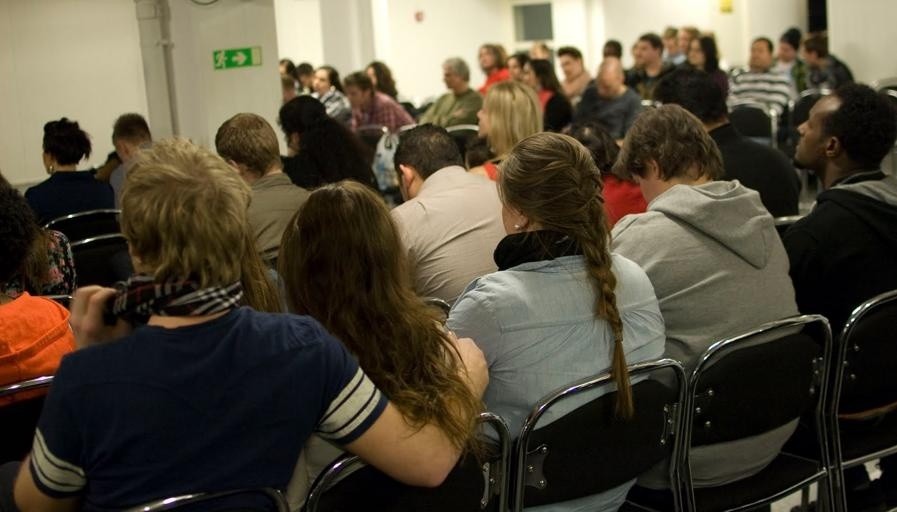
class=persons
[1,176,75,405]
[283,181,446,511]
[23,114,312,310]
[12,140,490,511]
[450,134,666,511]
[282,22,896,311]
[776,84,897,511]
[605,104,804,490]
